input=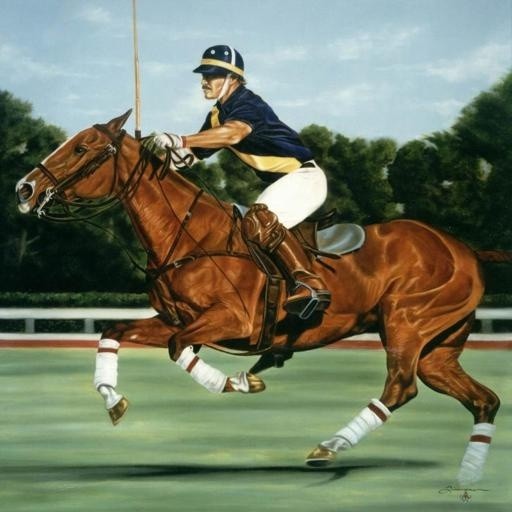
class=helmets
[193,44,246,83]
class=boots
[275,230,330,309]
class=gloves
[153,133,183,151]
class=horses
[13,108,512,488]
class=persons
[149,45,331,314]
